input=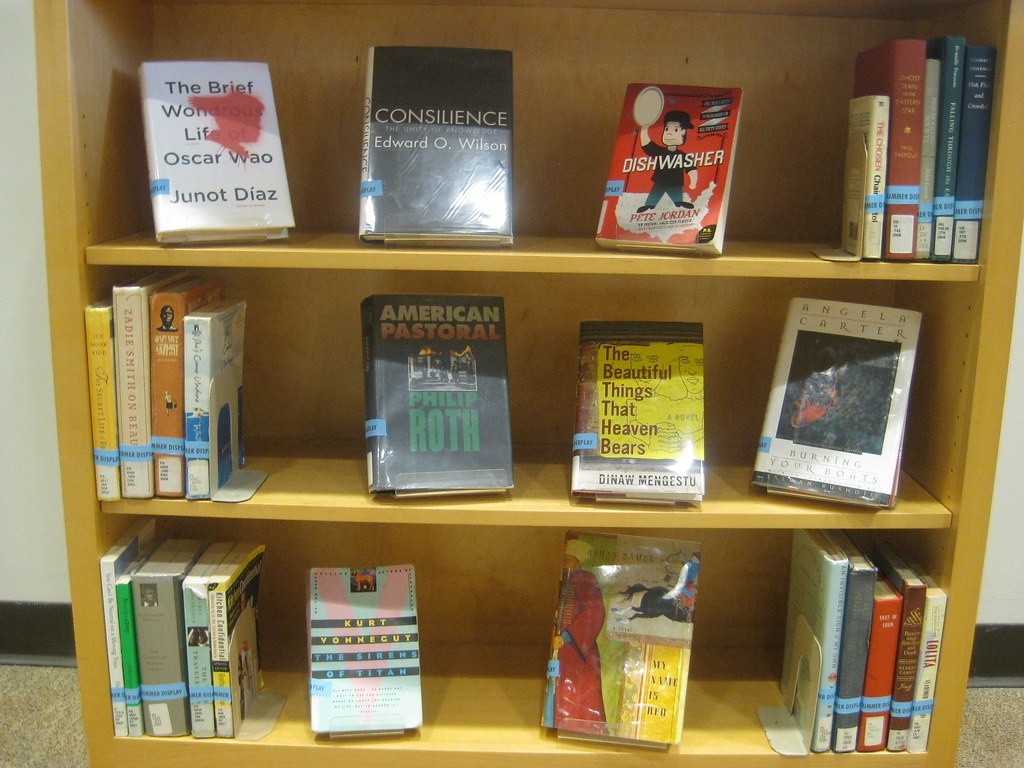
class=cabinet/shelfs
[34,0,1024,768]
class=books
[569,319,706,501]
[99,518,265,738]
[595,82,741,255]
[85,268,246,503]
[360,45,516,247]
[139,59,296,242]
[840,35,998,266]
[778,527,948,752]
[360,293,516,494]
[306,564,421,732]
[541,531,702,744]
[750,298,924,509]
